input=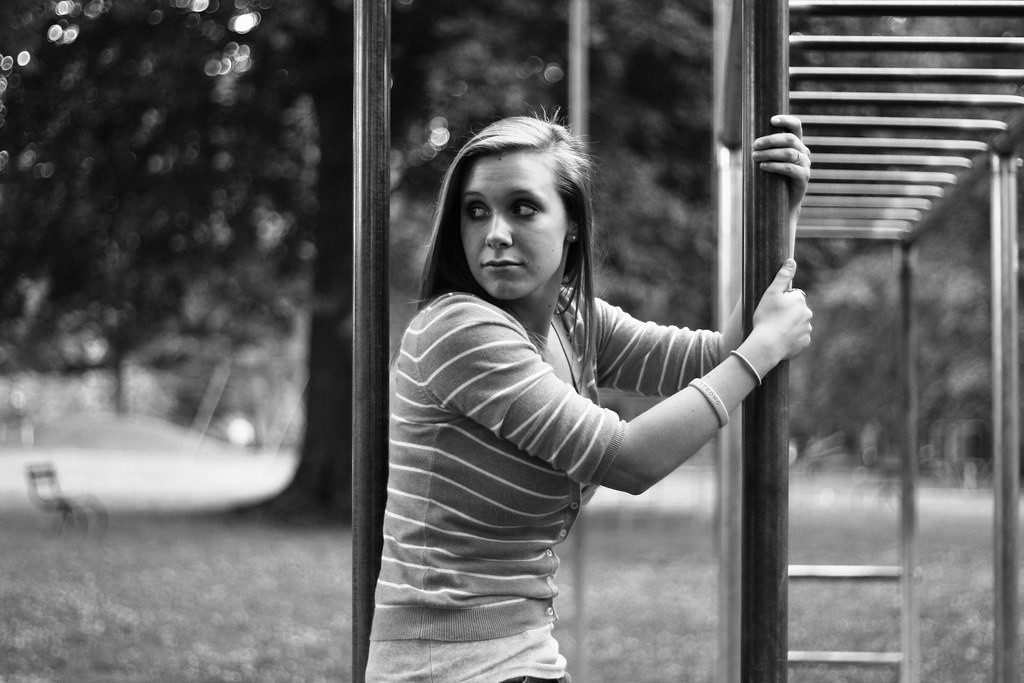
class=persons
[364,112,814,683]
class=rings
[791,288,806,297]
[794,150,800,164]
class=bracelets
[689,378,729,428]
[730,350,761,386]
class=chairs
[26,463,109,541]
